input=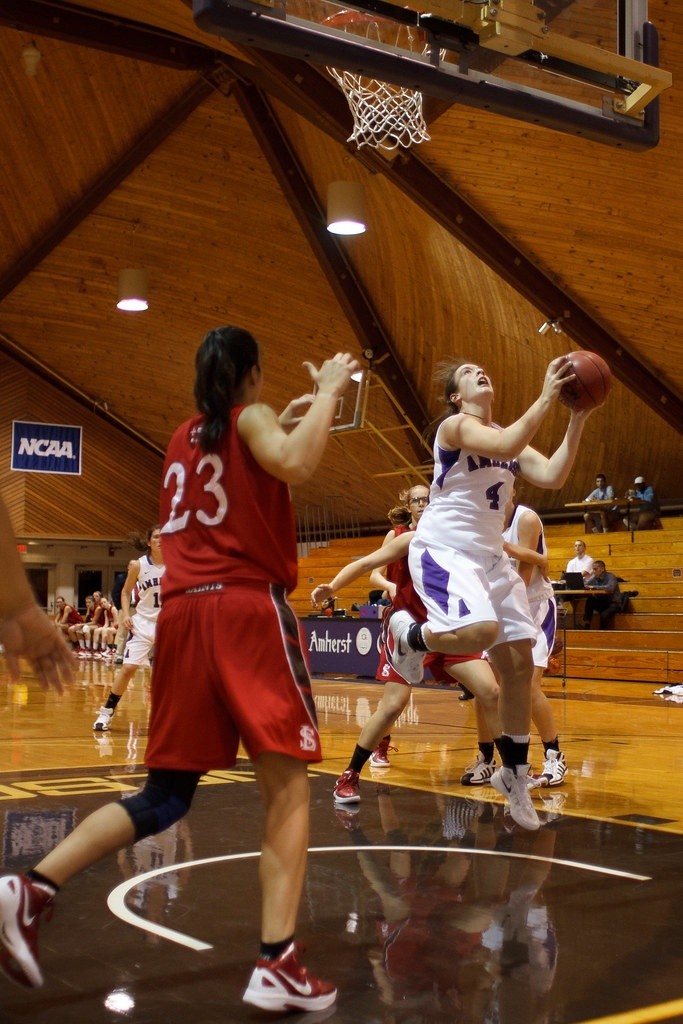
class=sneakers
[490,761,540,830]
[91,705,114,731]
[383,609,426,685]
[332,768,362,804]
[73,648,123,663]
[92,731,113,746]
[460,750,497,785]
[369,737,399,767]
[538,747,568,788]
[333,801,360,832]
[0,872,57,992]
[242,941,337,1012]
[524,763,548,789]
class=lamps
[327,156,368,236]
[116,220,151,312]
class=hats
[634,477,646,484]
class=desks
[554,590,613,630]
[297,617,434,680]
[565,499,648,531]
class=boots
[459,682,475,701]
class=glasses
[408,496,428,506]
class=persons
[53,591,120,659]
[561,539,596,584]
[0,324,361,1012]
[320,596,333,616]
[311,357,605,829]
[94,525,165,731]
[334,786,558,1024]
[623,477,659,530]
[114,560,140,665]
[576,561,621,630]
[0,498,79,697]
[583,474,614,531]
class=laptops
[564,572,597,591]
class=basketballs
[557,350,613,413]
